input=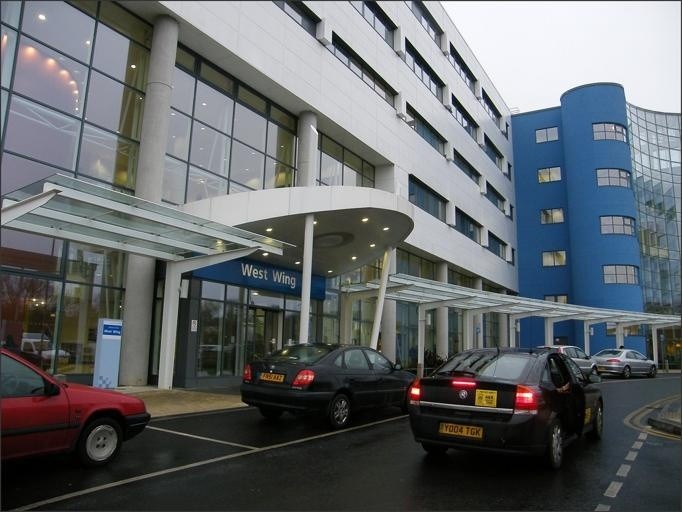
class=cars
[21,333,95,360]
[0,346,151,471]
[536,345,600,380]
[590,348,658,377]
[407,346,604,472]
[240,343,418,431]
